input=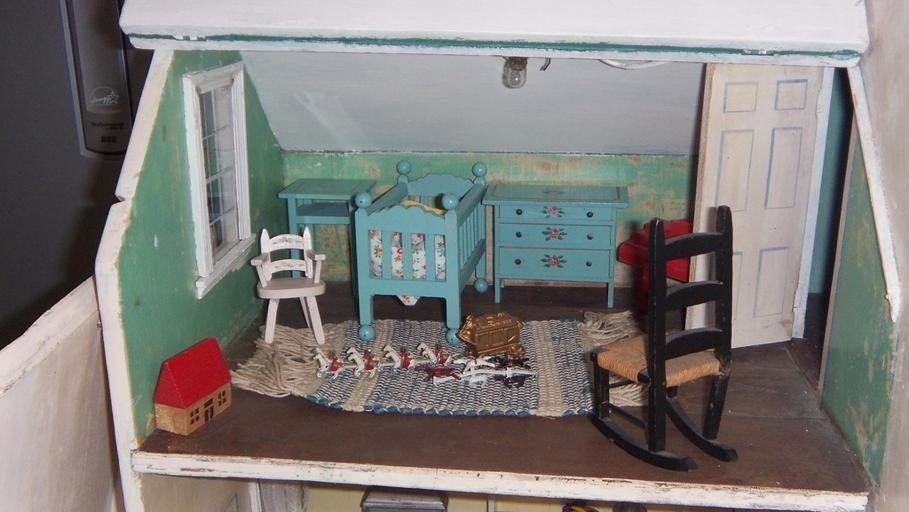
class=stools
[248,226,330,349]
[593,203,739,473]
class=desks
[276,178,376,294]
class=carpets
[229,316,673,421]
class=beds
[352,160,488,345]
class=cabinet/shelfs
[478,182,628,310]
